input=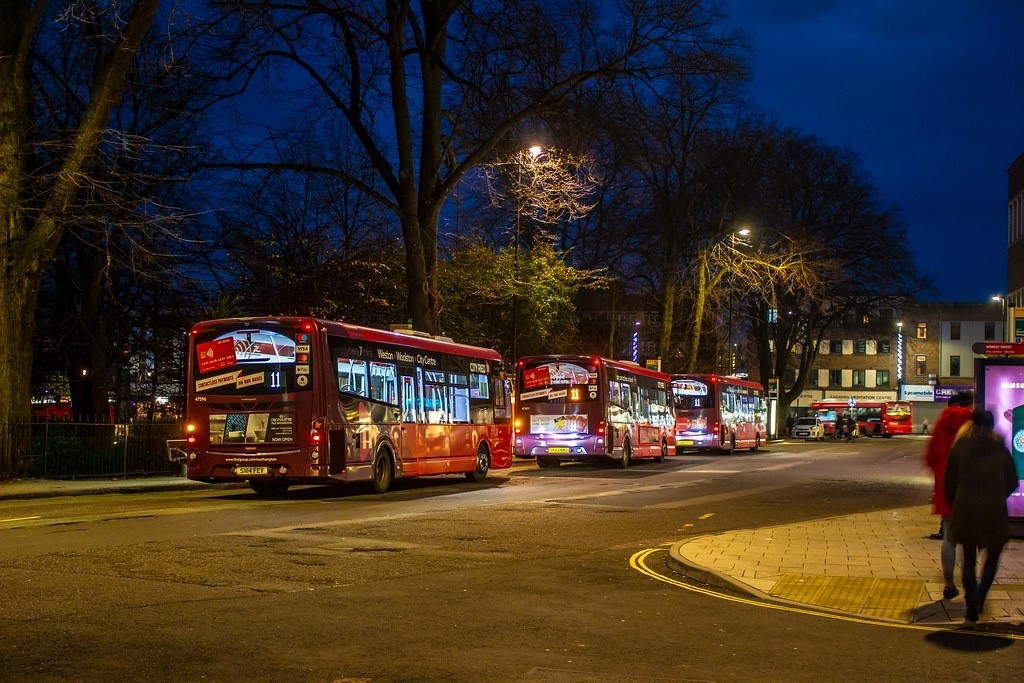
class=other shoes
[941,586,960,601]
[964,597,987,622]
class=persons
[947,395,961,409]
[924,390,976,540]
[785,413,794,437]
[941,408,1019,631]
[872,409,878,418]
[922,415,930,437]
[836,411,859,443]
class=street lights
[896,320,907,404]
[508,142,541,377]
[724,225,751,375]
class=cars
[792,416,825,440]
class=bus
[186,315,513,497]
[809,400,913,439]
[668,374,766,454]
[513,353,677,469]
